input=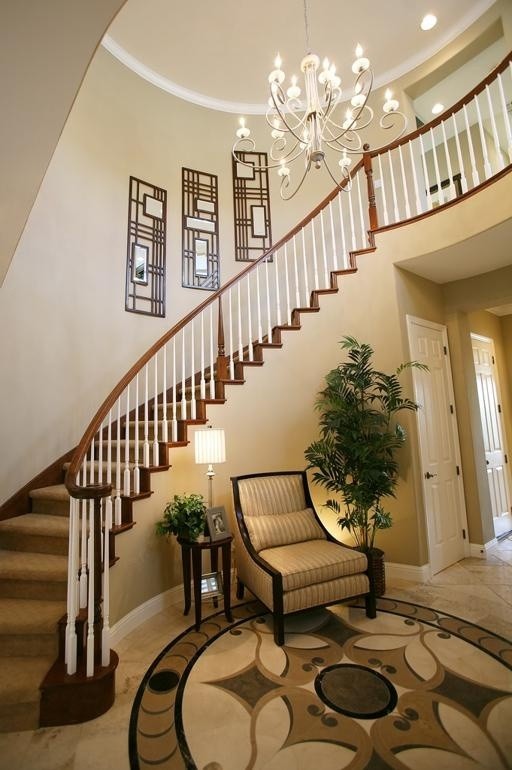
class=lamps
[192,424,228,509]
[231,3,414,200]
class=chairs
[227,470,376,647]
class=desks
[174,535,235,633]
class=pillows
[242,506,328,555]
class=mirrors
[193,196,217,215]
[183,214,218,236]
[142,193,165,223]
[129,241,150,287]
[249,203,268,240]
[192,238,209,279]
[233,159,255,182]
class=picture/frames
[204,503,233,544]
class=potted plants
[301,329,432,599]
[153,490,207,544]
